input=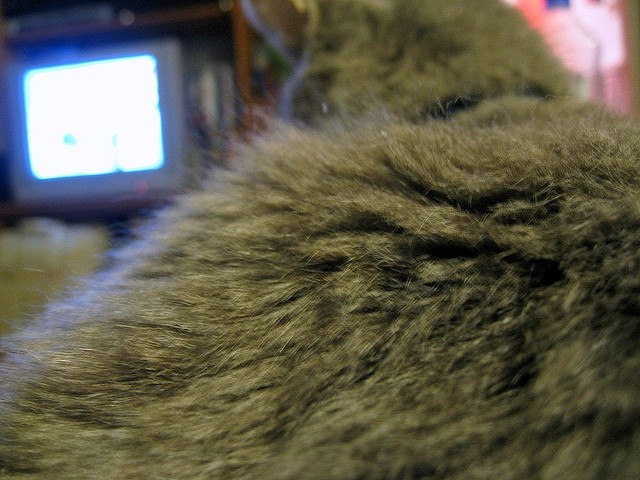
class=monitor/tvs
[4,41,190,203]
